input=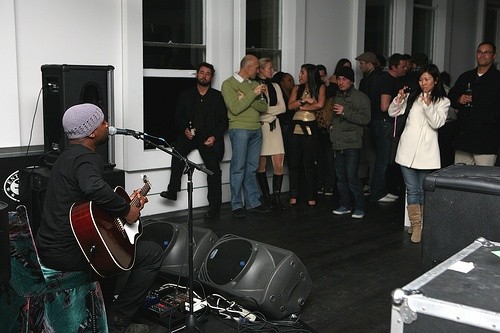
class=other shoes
[352,209,365,219]
[363,185,371,195]
[247,204,273,213]
[316,184,334,196]
[378,192,399,203]
[232,208,246,218]
[107,314,149,333]
[333,206,352,215]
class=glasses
[477,50,492,55]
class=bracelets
[300,98,304,105]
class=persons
[316,65,328,84]
[356,51,382,196]
[255,57,289,212]
[162,62,229,218]
[222,55,272,218]
[274,69,307,194]
[329,58,352,84]
[388,63,452,243]
[367,54,409,202]
[36,104,163,333]
[448,42,500,167]
[287,63,326,209]
[329,65,371,218]
[404,53,454,173]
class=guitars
[69,174,151,277]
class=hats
[62,103,105,140]
[336,66,355,83]
[355,51,378,64]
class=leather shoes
[203,209,221,218]
[159,191,178,201]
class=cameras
[404,86,415,93]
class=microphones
[108,126,145,136]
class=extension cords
[232,305,257,322]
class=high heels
[308,200,316,208]
[289,199,297,208]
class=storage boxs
[390,236,500,333]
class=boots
[257,172,274,208]
[407,204,423,243]
[272,173,287,211]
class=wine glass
[259,80,266,102]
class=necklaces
[304,87,310,96]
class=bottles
[465,83,473,107]
[335,91,342,106]
[188,121,195,138]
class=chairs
[9,205,108,333]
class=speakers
[23,65,125,227]
[198,233,312,320]
[141,218,218,282]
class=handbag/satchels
[312,82,335,129]
